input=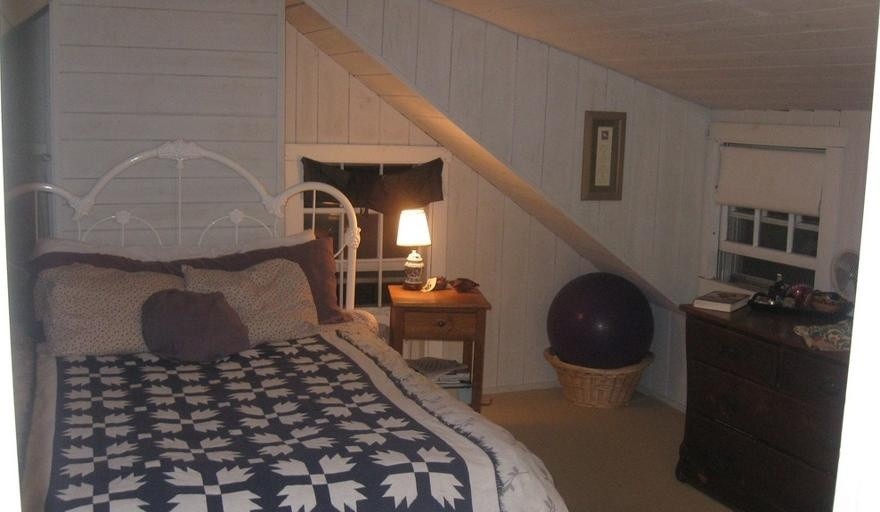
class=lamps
[397,210,431,290]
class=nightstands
[387,285,491,415]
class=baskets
[544,347,656,410]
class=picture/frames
[580,110,627,201]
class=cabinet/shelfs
[676,303,854,512]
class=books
[692,290,752,313]
[404,356,470,384]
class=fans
[832,249,859,303]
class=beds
[5,139,569,512]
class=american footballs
[810,293,852,313]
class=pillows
[27,247,171,341]
[139,285,252,363]
[179,257,322,348]
[30,261,182,359]
[173,235,353,325]
